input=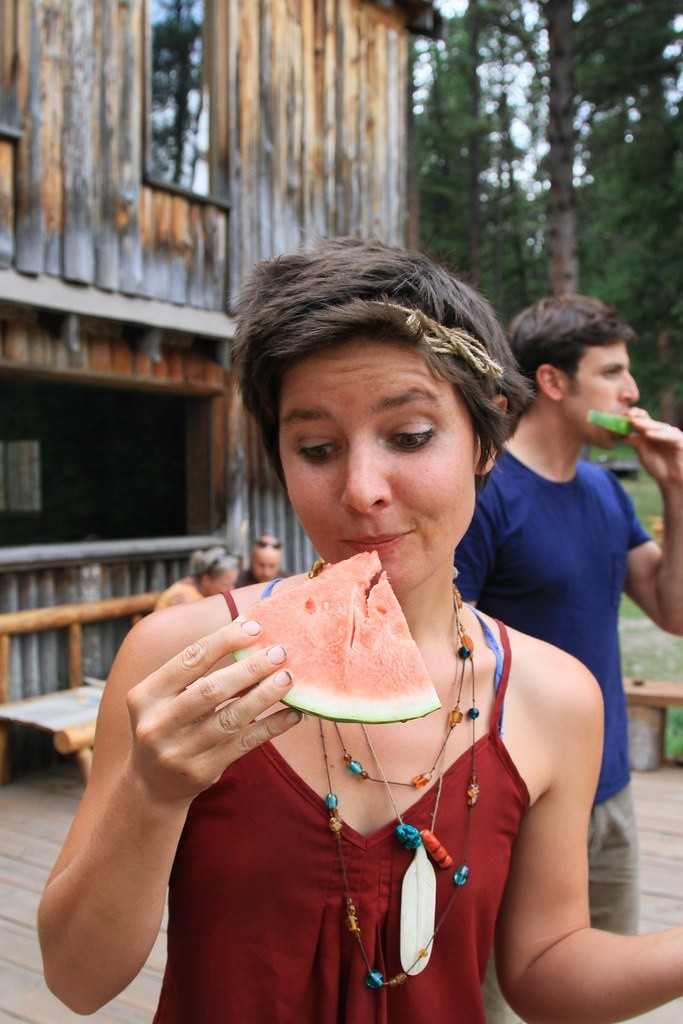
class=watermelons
[227,550,442,725]
[586,409,638,436]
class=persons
[155,547,237,612]
[234,536,294,589]
[452,298,683,1024]
[39,239,683,1024]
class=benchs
[0,591,163,786]
[624,678,683,770]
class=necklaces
[306,556,478,991]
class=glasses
[255,540,282,549]
